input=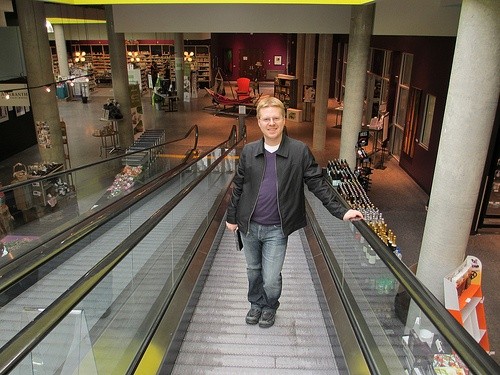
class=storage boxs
[286,108,303,122]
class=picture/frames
[273,55,282,66]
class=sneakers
[258,306,276,328]
[245,304,262,324]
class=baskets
[13,163,28,181]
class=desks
[335,107,343,129]
[164,95,179,112]
[303,100,315,122]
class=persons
[151,61,160,89]
[226,97,363,328]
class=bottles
[327,158,403,260]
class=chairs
[235,78,253,101]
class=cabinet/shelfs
[274,77,299,109]
[85,53,212,91]
[27,165,63,207]
[444,254,493,357]
[92,110,123,159]
